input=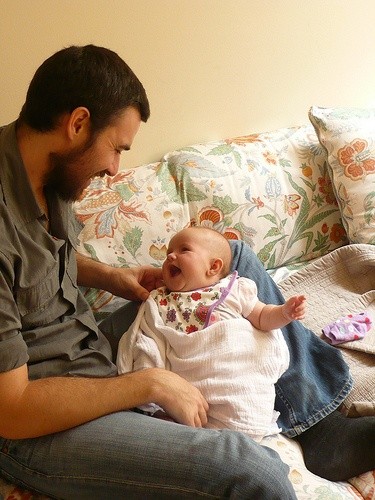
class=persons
[117,227,307,444]
[0,45,375,500]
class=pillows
[308,104,375,247]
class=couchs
[71,103,375,500]
